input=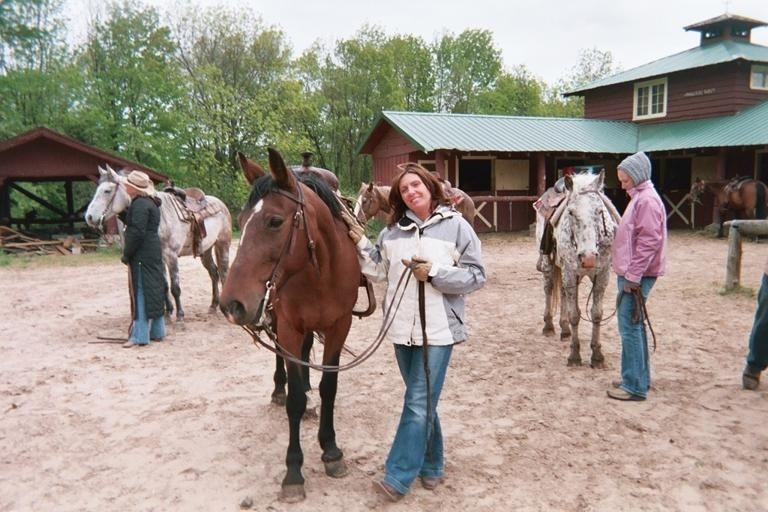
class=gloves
[120,255,130,265]
[401,255,433,283]
[341,212,365,244]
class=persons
[606,151,668,401]
[340,163,487,503]
[116,169,166,348]
[742,260,768,390]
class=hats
[617,151,653,187]
[121,170,154,195]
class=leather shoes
[743,365,761,390]
[122,341,147,350]
[607,388,647,401]
[612,380,650,391]
[371,479,402,502]
[423,476,441,491]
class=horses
[690,177,768,238]
[218,148,362,503]
[85,163,233,324]
[352,181,475,230]
[543,167,616,369]
